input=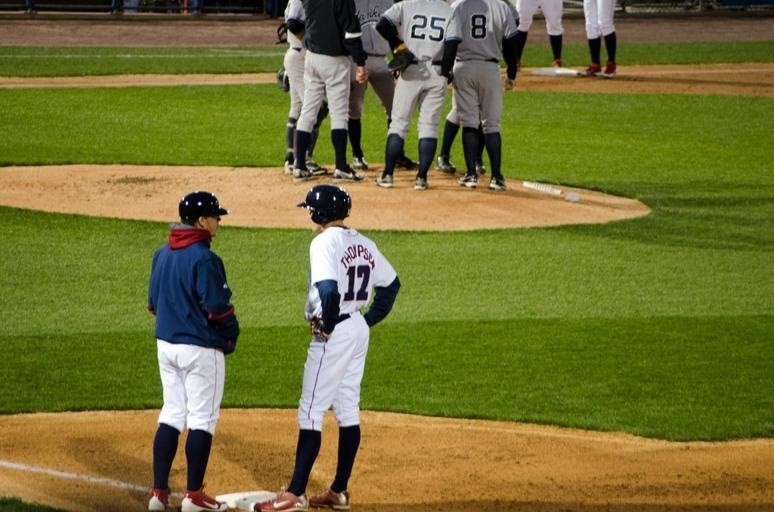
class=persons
[147,190,240,511]
[515,0,563,69]
[253,184,400,511]
[281,1,521,190]
[578,1,616,77]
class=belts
[338,315,349,322]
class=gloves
[310,319,331,341]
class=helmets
[297,184,352,212]
[179,192,228,223]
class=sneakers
[283,156,506,193]
[254,493,310,512]
[181,491,229,511]
[148,488,174,510]
[578,62,602,76]
[310,489,350,510]
[603,61,616,78]
